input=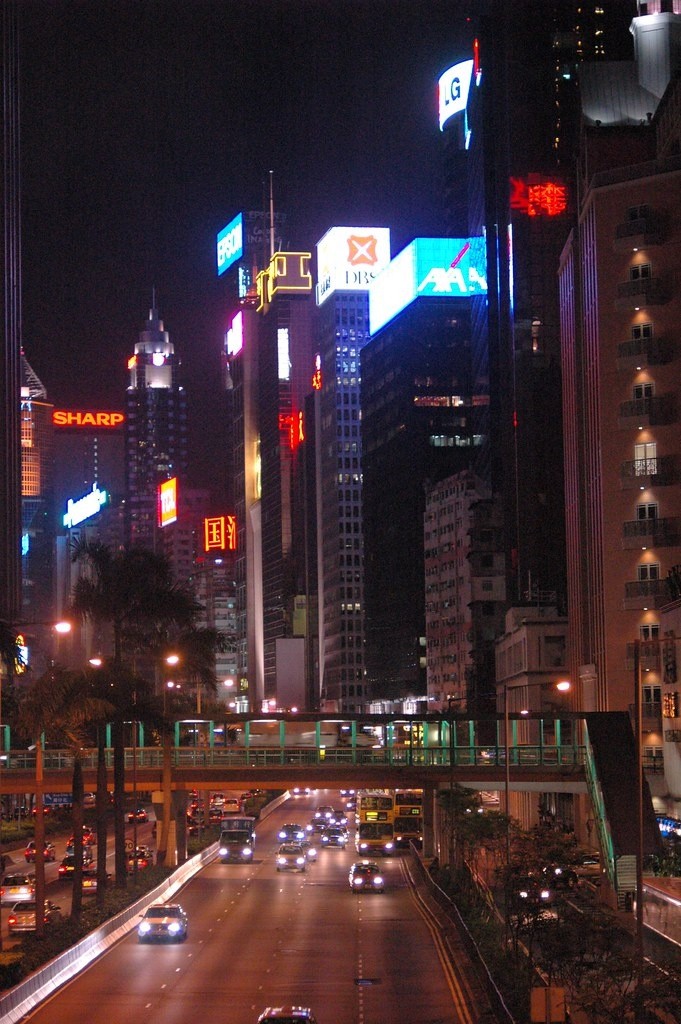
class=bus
[392,788,423,847]
[356,794,395,857]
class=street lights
[113,608,178,891]
[502,677,569,870]
[254,698,302,718]
[5,619,102,723]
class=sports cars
[4,793,119,824]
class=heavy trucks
[188,721,382,766]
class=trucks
[219,817,257,865]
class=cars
[24,840,56,862]
[278,784,356,861]
[1,872,38,906]
[258,1007,318,1024]
[8,898,61,937]
[60,825,156,897]
[127,789,265,837]
[349,861,385,894]
[275,844,305,872]
[461,798,592,954]
[137,903,188,945]
